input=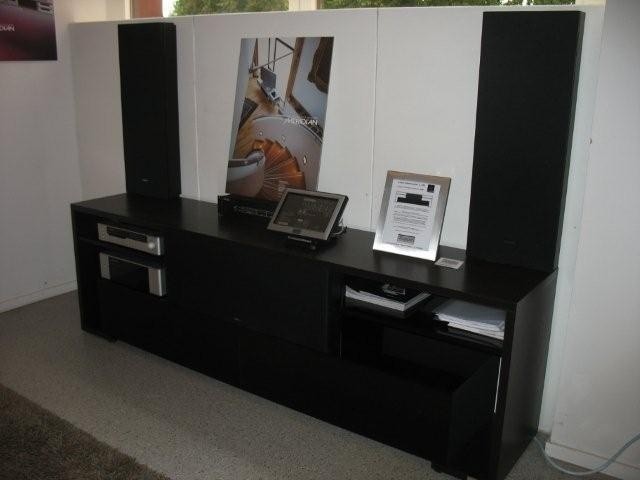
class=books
[343,280,425,313]
[433,300,504,340]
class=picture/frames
[372,170,451,261]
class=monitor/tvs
[266,187,347,246]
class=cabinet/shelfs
[166,234,327,426]
[327,257,554,480]
[71,205,164,362]
